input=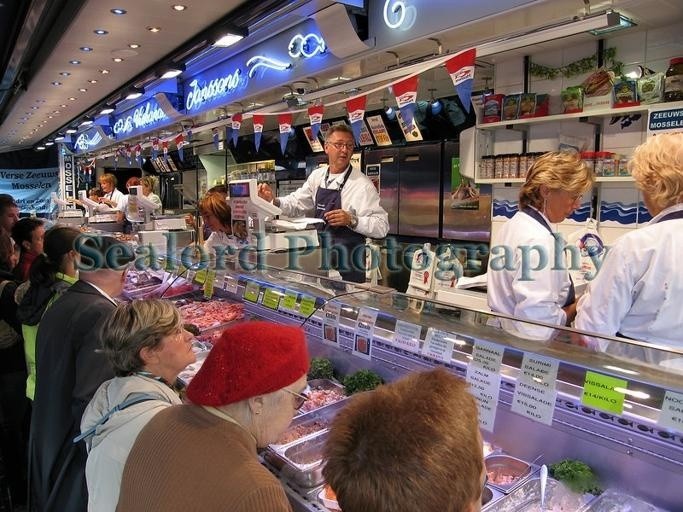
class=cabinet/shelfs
[459,103,682,226]
[39,220,683,512]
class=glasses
[325,141,355,149]
[148,319,195,350]
[282,387,310,409]
[550,187,586,207]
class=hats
[186,321,307,407]
[72,235,137,272]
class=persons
[1,195,20,247]
[116,177,160,222]
[1,228,24,377]
[78,300,197,512]
[488,151,594,343]
[115,322,310,512]
[258,124,390,283]
[571,133,682,355]
[69,190,91,217]
[321,368,487,512]
[185,186,227,246]
[15,217,46,274]
[201,184,254,255]
[88,174,123,213]
[25,236,134,512]
[139,176,162,217]
[19,227,82,420]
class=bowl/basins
[295,376,352,414]
[582,487,662,512]
[483,447,542,494]
[273,427,327,472]
[478,475,593,512]
[481,483,503,510]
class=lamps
[44,138,54,146]
[100,102,114,115]
[54,132,65,141]
[156,58,187,79]
[213,23,249,48]
[77,116,94,127]
[65,127,77,135]
[124,84,143,100]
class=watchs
[349,214,356,228]
